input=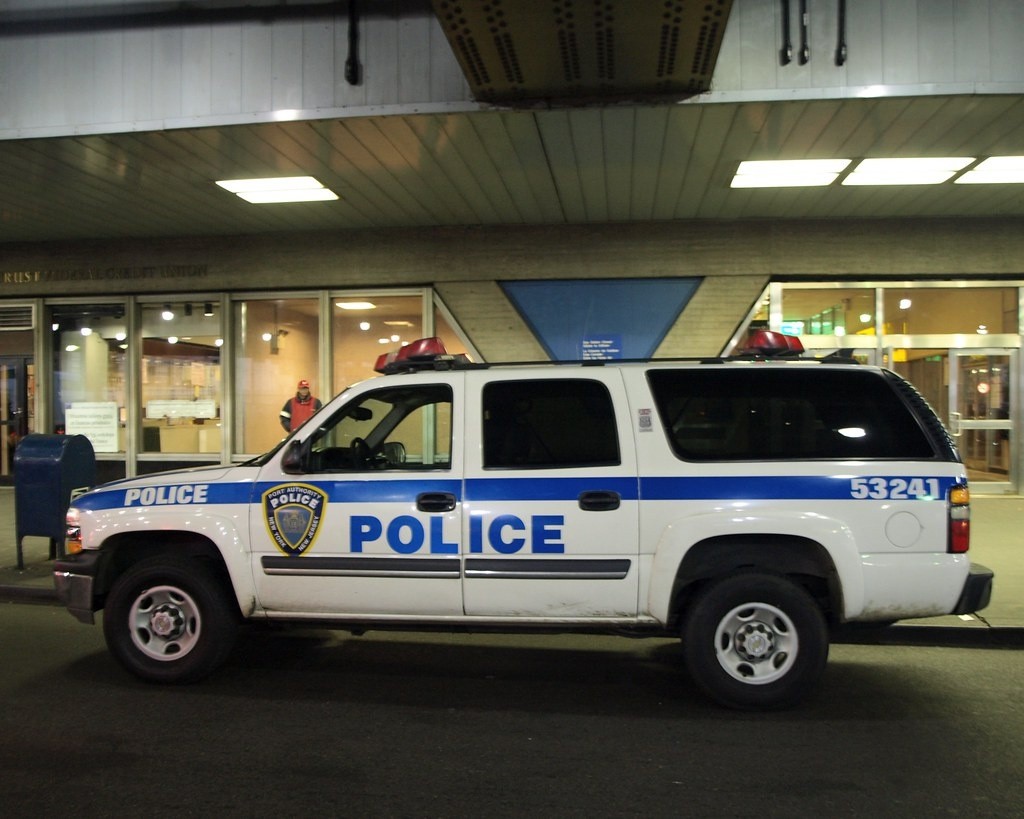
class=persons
[280,379,325,445]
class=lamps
[161,303,174,321]
[203,302,215,318]
[183,301,194,316]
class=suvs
[51,327,996,716]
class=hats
[298,380,310,388]
